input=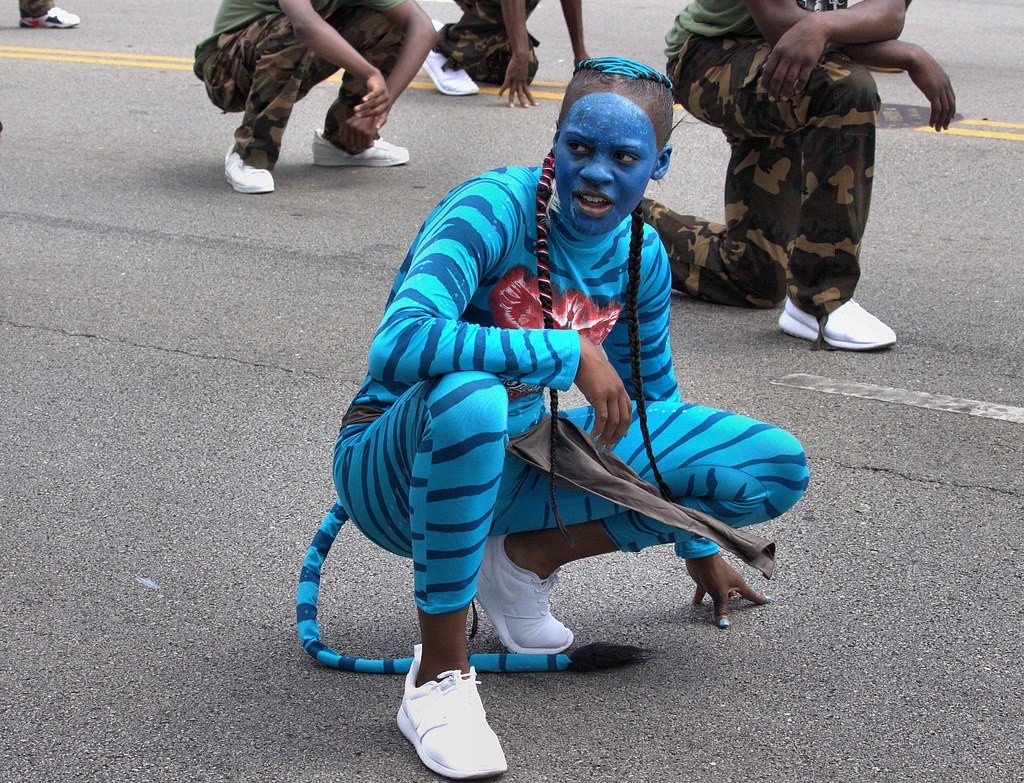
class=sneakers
[475,534,575,656]
[221,144,276,194]
[308,128,410,167]
[17,7,82,28]
[778,294,898,350]
[394,643,509,779]
[424,17,480,96]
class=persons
[191,0,439,195]
[584,1,957,351]
[18,1,79,27]
[296,56,809,779]
[423,0,589,108]
